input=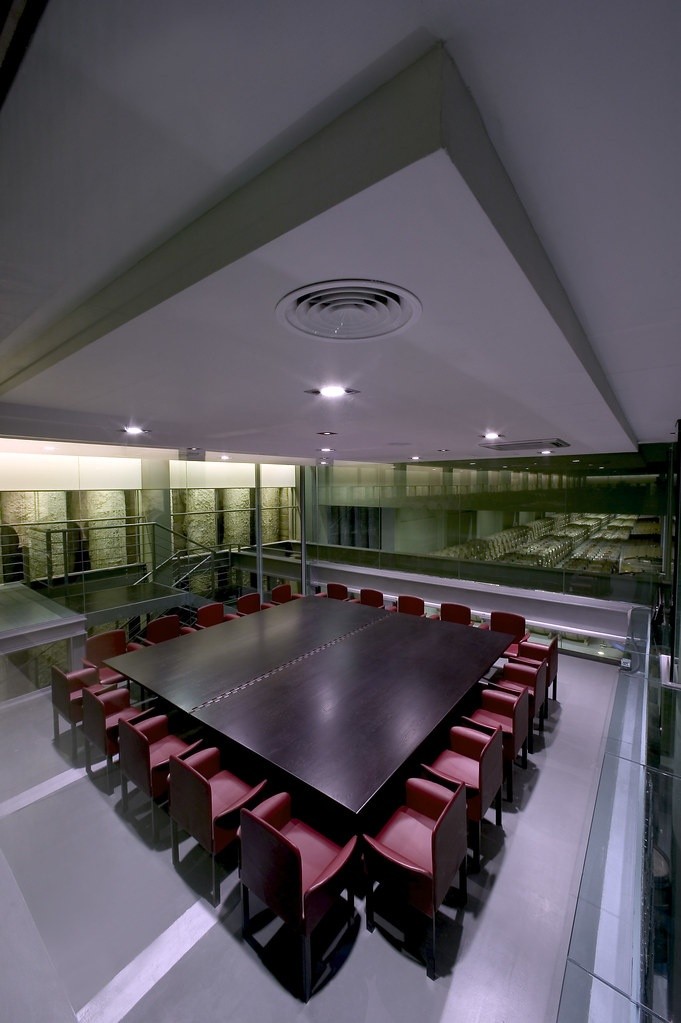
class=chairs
[237,592,275,617]
[462,687,528,803]
[365,777,469,979]
[142,615,197,645]
[52,666,118,764]
[117,714,204,843]
[237,792,359,1003]
[509,636,560,718]
[194,603,241,629]
[348,588,386,609]
[270,585,304,605]
[167,745,271,906]
[420,725,504,873]
[81,688,156,796]
[314,582,351,601]
[428,603,475,627]
[84,629,146,697]
[478,610,530,658]
[385,594,427,618]
[489,657,547,754]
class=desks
[100,595,518,900]
